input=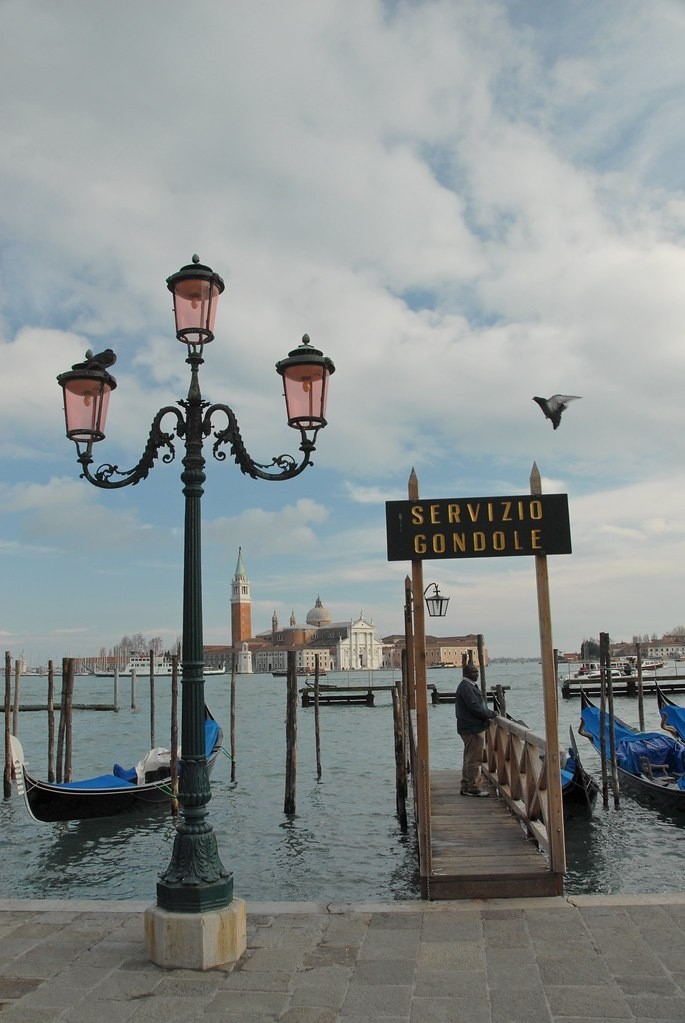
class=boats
[652,677,685,745]
[81,649,228,676]
[557,653,666,682]
[9,701,227,825]
[577,682,685,797]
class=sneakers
[460,790,467,795]
[467,790,489,797]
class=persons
[454,664,499,797]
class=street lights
[405,575,453,709]
[56,253,338,986]
